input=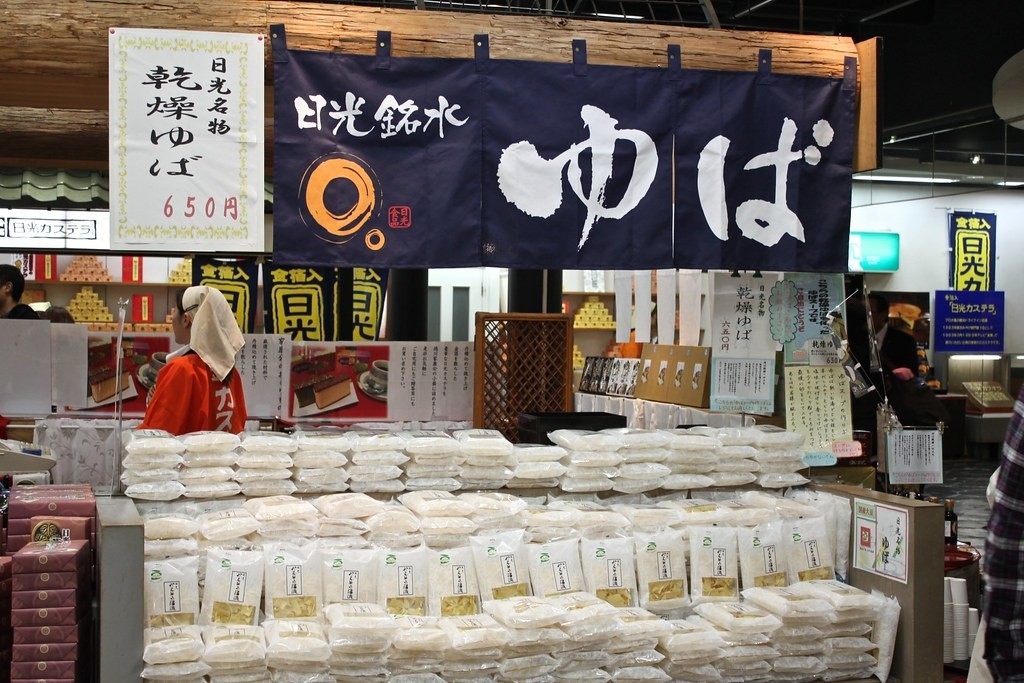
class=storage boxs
[0,484,96,683]
[517,411,626,445]
[578,343,711,407]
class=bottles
[895,492,958,545]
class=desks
[574,391,786,438]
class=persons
[133,283,248,435]
[983,382,1024,683]
[0,264,40,320]
[41,305,78,324]
[866,293,919,423]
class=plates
[136,363,151,390]
[357,371,387,401]
[292,381,358,416]
[69,374,138,410]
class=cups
[368,359,388,391]
[148,352,168,381]
[944,576,980,662]
